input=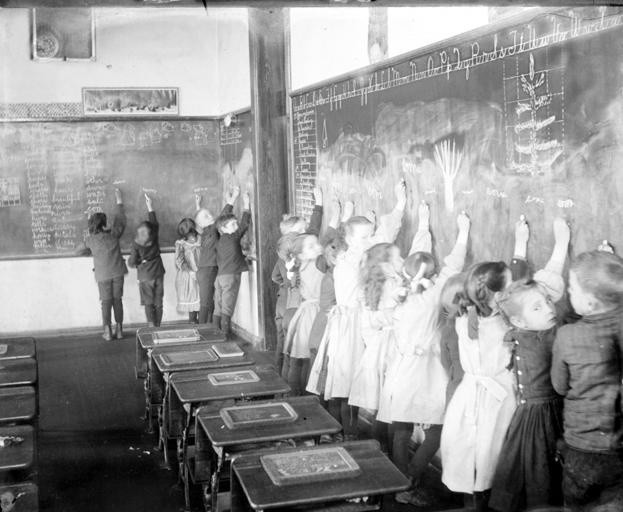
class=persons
[273,180,622,511]
[175,194,203,322]
[195,185,240,325]
[75,189,128,342]
[128,197,165,328]
[213,191,250,333]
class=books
[212,340,244,360]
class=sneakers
[396,488,430,507]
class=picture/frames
[83,86,180,117]
[32,8,96,61]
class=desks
[0,336,40,512]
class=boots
[102,325,113,341]
[213,315,223,335]
[221,315,237,342]
[112,322,124,339]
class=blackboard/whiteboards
[1,107,255,261]
[283,6,623,271]
